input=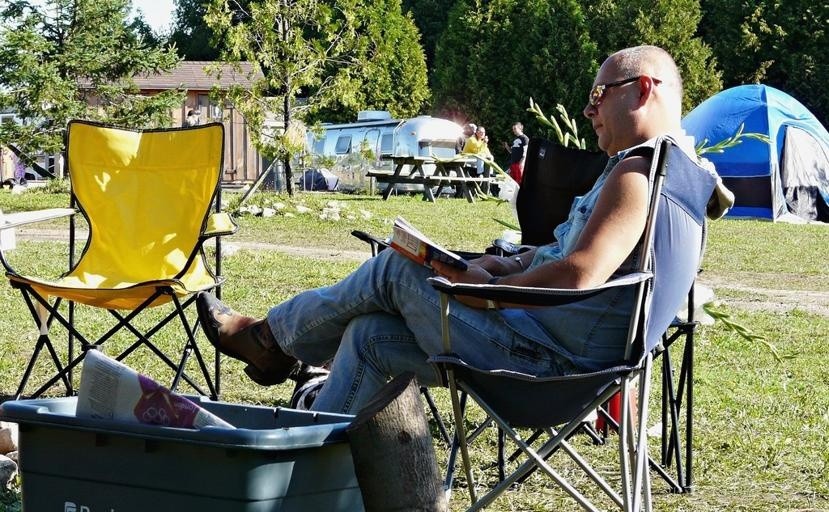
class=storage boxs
[0,394,366,512]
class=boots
[196,291,299,386]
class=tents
[680,82,829,223]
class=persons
[185,109,202,127]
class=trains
[293,110,482,196]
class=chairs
[347,134,720,511]
[0,122,240,402]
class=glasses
[588,76,664,106]
